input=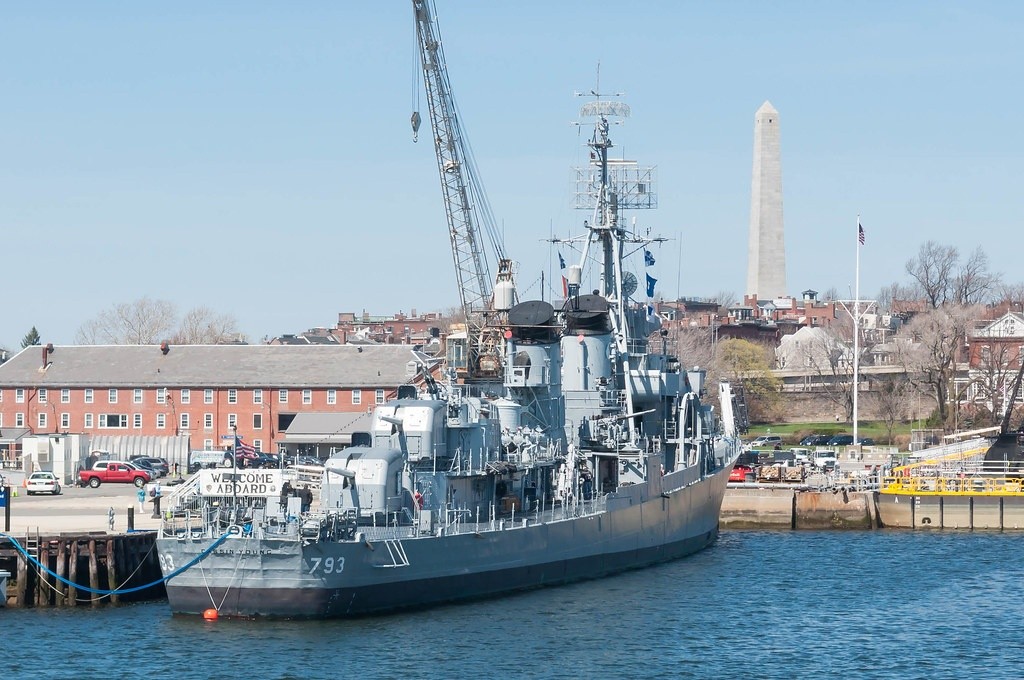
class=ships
[150,0,755,622]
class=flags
[236,436,259,461]
[558,248,657,297]
[859,224,866,246]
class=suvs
[91,456,169,480]
[728,444,841,481]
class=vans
[751,434,783,447]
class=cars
[848,438,875,445]
[23,471,61,495]
[826,435,854,446]
[798,434,832,446]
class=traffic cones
[22,479,27,488]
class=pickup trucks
[79,463,152,488]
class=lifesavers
[413,493,423,510]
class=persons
[299,485,313,513]
[138,485,156,513]
[107,507,115,530]
